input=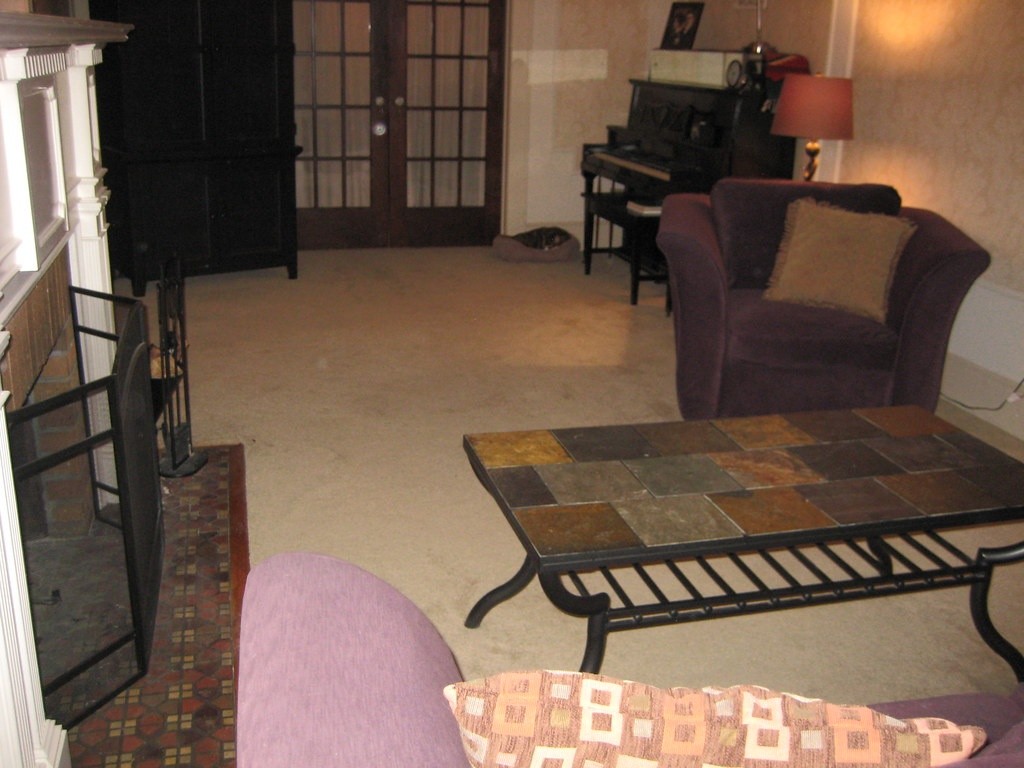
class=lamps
[770,75,856,182]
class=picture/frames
[659,2,705,49]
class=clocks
[725,60,743,96]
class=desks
[464,402,1024,685]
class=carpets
[21,441,251,768]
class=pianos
[581,77,796,318]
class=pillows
[762,197,919,323]
[443,669,991,768]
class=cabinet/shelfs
[86,0,305,299]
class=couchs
[236,552,1024,768]
[654,177,993,422]
